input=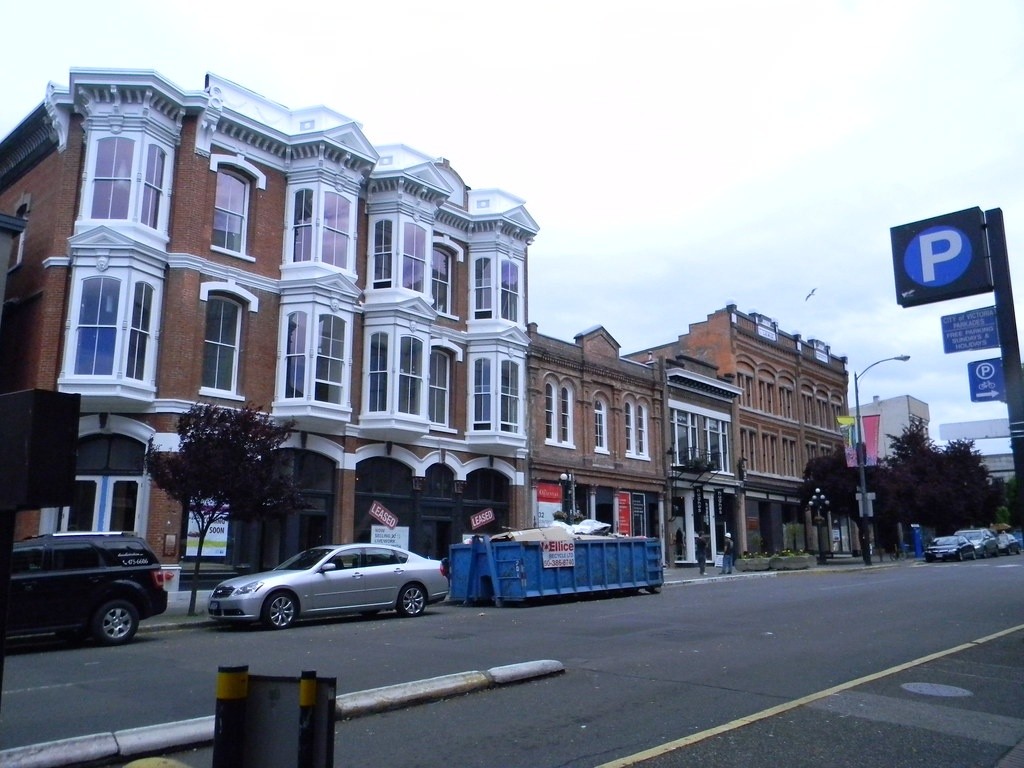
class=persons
[720,532,734,574]
[696,531,708,576]
[676,528,685,560]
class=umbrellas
[989,523,1012,532]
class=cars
[954,528,999,558]
[924,536,976,563]
[207,543,449,630]
[998,531,1021,555]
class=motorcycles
[890,542,909,561]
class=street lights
[558,471,575,524]
[808,488,831,565]
[854,356,910,566]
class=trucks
[683,531,699,553]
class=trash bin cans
[446,535,665,608]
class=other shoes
[727,572,731,573]
[719,572,725,575]
[701,572,708,576]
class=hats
[724,533,731,538]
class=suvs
[6,531,168,645]
